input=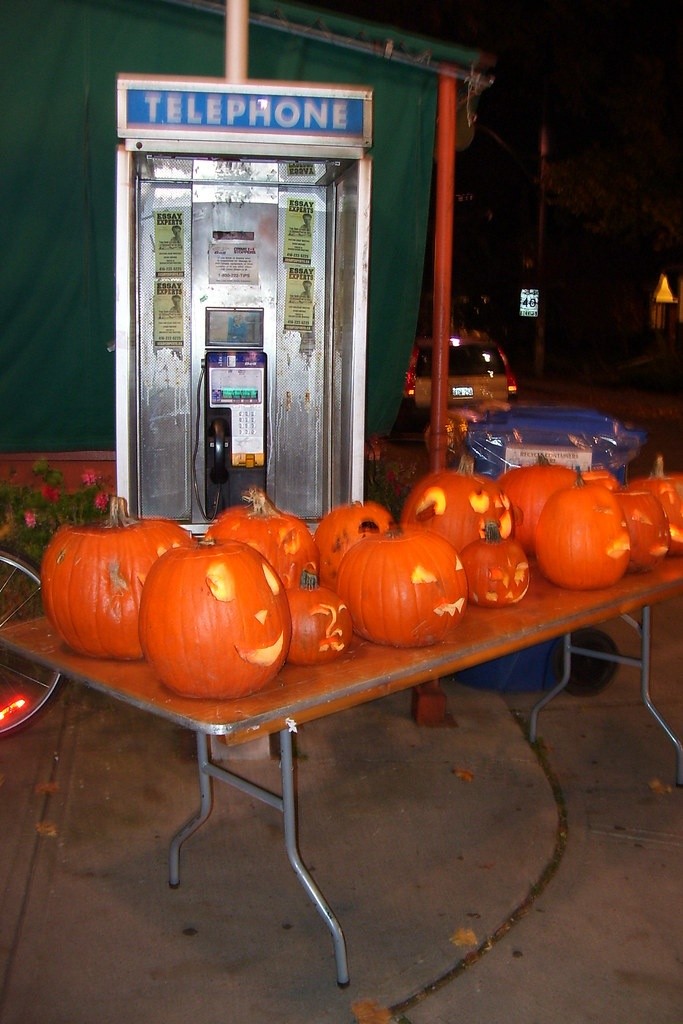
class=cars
[386,332,516,434]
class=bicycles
[1,545,76,742]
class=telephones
[208,418,231,484]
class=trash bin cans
[456,404,646,696]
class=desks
[0,553,682,986]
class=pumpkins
[496,454,585,557]
